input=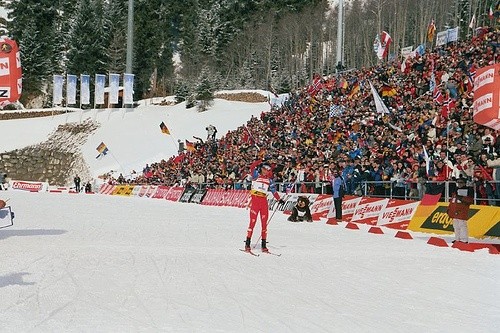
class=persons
[332,168,347,221]
[85,181,91,192]
[99,23,500,205]
[74,174,81,192]
[243,157,284,251]
[447,177,476,243]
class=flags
[433,86,444,105]
[185,139,197,152]
[314,75,323,87]
[51,74,134,125]
[96,142,108,156]
[423,145,432,177]
[241,124,248,142]
[160,121,170,134]
[441,93,455,118]
[468,73,474,91]
[307,86,317,96]
[373,33,387,59]
[330,100,341,117]
[467,0,500,28]
[340,75,348,89]
[381,30,392,49]
[383,82,397,97]
[429,71,436,92]
[370,82,390,113]
[427,22,434,41]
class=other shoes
[262,248,268,252]
[336,219,341,222]
[245,246,251,252]
[452,240,458,243]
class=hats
[484,137,491,141]
[474,168,482,174]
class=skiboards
[238,246,282,258]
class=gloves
[279,199,285,205]
